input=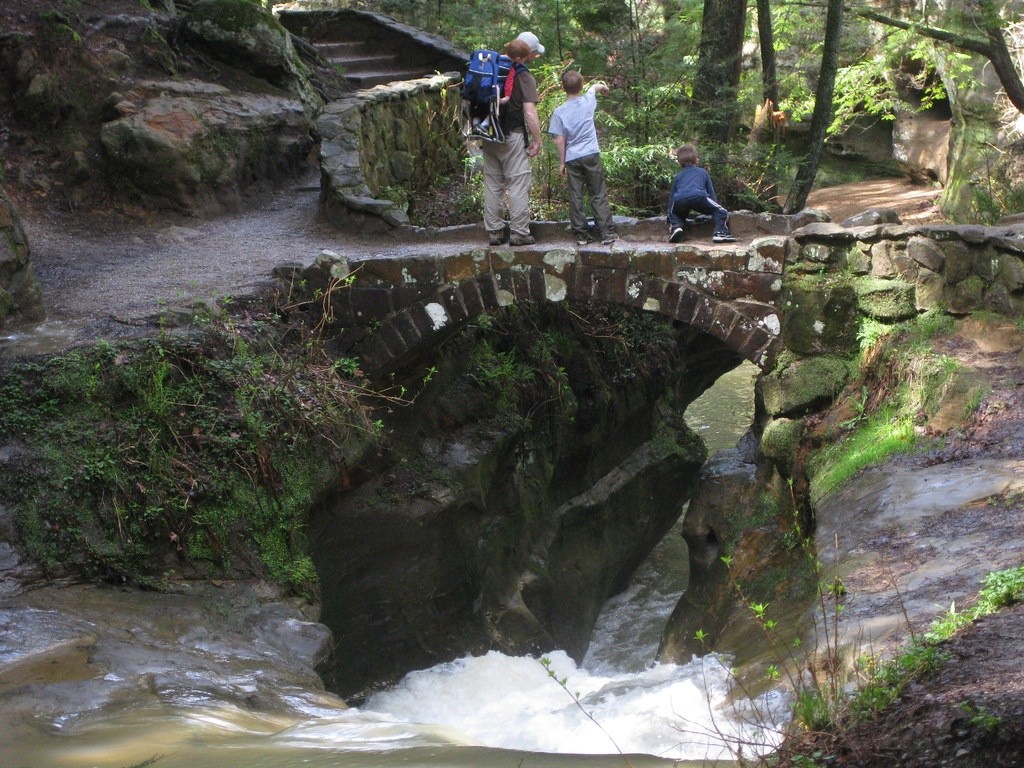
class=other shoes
[473,124,492,137]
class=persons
[482,32,546,245]
[666,144,736,242]
[472,40,530,135]
[548,70,619,245]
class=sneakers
[577,233,593,244]
[489,229,508,245]
[669,227,683,242]
[601,232,619,245]
[509,231,535,246]
[713,232,736,243]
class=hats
[517,31,545,53]
